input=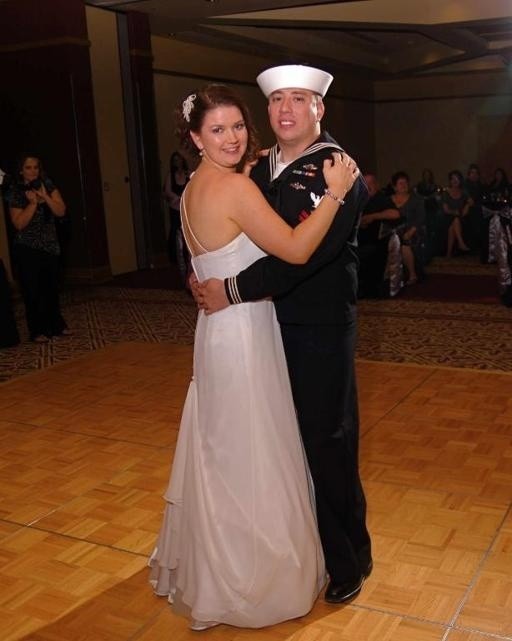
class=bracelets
[322,188,345,206]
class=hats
[256,64,334,99]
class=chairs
[169,207,194,296]
[356,185,512,307]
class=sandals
[33,332,74,344]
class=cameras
[23,178,43,192]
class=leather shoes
[325,557,373,603]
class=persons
[188,63,377,604]
[161,149,191,266]
[356,161,511,309]
[6,151,77,344]
[0,169,22,351]
[175,79,362,632]
